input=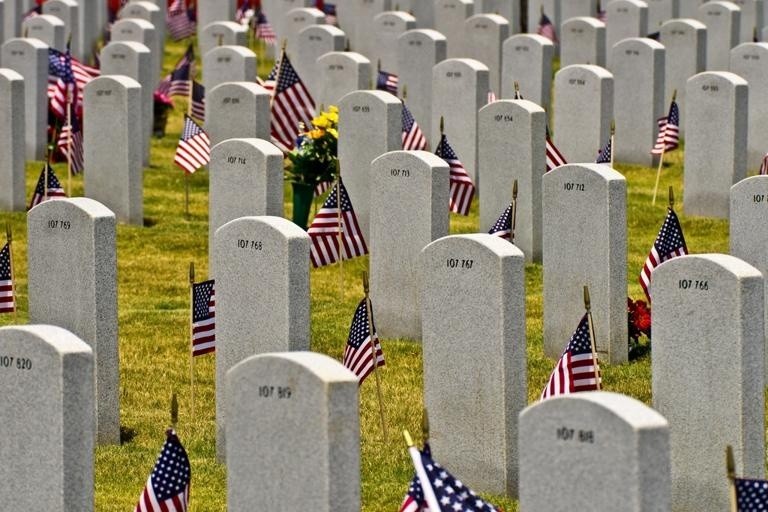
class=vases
[290,180,314,231]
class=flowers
[626,297,651,356]
[284,107,341,187]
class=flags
[2,0,690,355]
[541,314,602,400]
[343,297,385,387]
[402,452,497,511]
[734,479,768,511]
[137,431,191,511]
[758,155,768,176]
